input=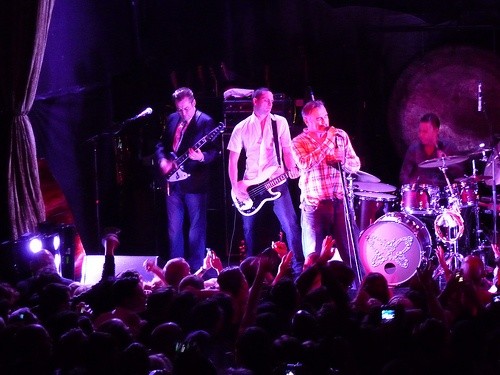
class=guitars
[230,165,300,217]
[126,121,227,201]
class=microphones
[325,125,343,135]
[135,107,153,118]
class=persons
[154,87,217,272]
[402,112,465,187]
[0,231,500,375]
[227,88,304,277]
[289,101,366,288]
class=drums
[442,181,481,211]
[351,181,398,197]
[352,190,401,233]
[357,211,433,286]
[394,183,441,217]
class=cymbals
[415,154,469,169]
[484,141,500,185]
[453,175,493,183]
[350,170,381,185]
[434,212,464,243]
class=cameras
[381,309,395,320]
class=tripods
[470,158,498,267]
